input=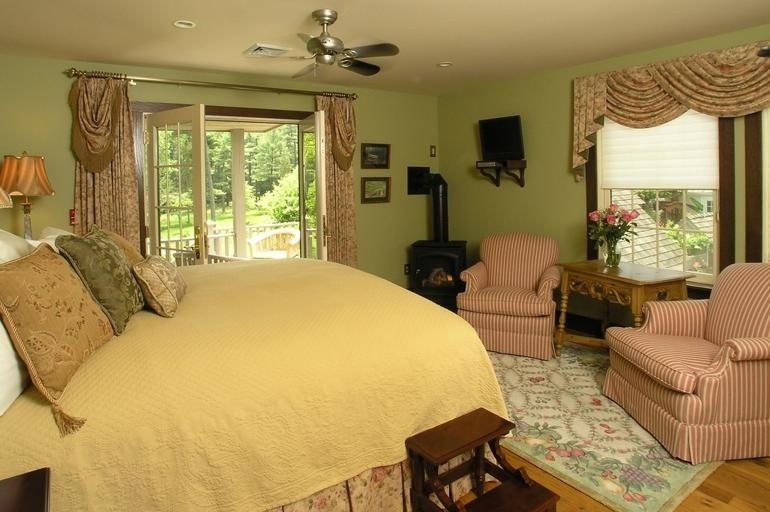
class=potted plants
[666,228,710,270]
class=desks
[556,259,695,358]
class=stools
[405,407,560,512]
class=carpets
[485,340,725,512]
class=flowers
[586,204,640,241]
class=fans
[260,8,399,78]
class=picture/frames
[361,177,391,204]
[361,143,391,169]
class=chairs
[602,262,770,465]
[457,233,561,360]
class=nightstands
[0,466,50,512]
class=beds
[0,231,512,512]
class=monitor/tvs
[478,115,525,161]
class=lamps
[0,151,57,240]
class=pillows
[0,243,114,437]
[104,227,144,268]
[55,224,145,337]
[133,254,186,318]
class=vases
[603,241,622,268]
